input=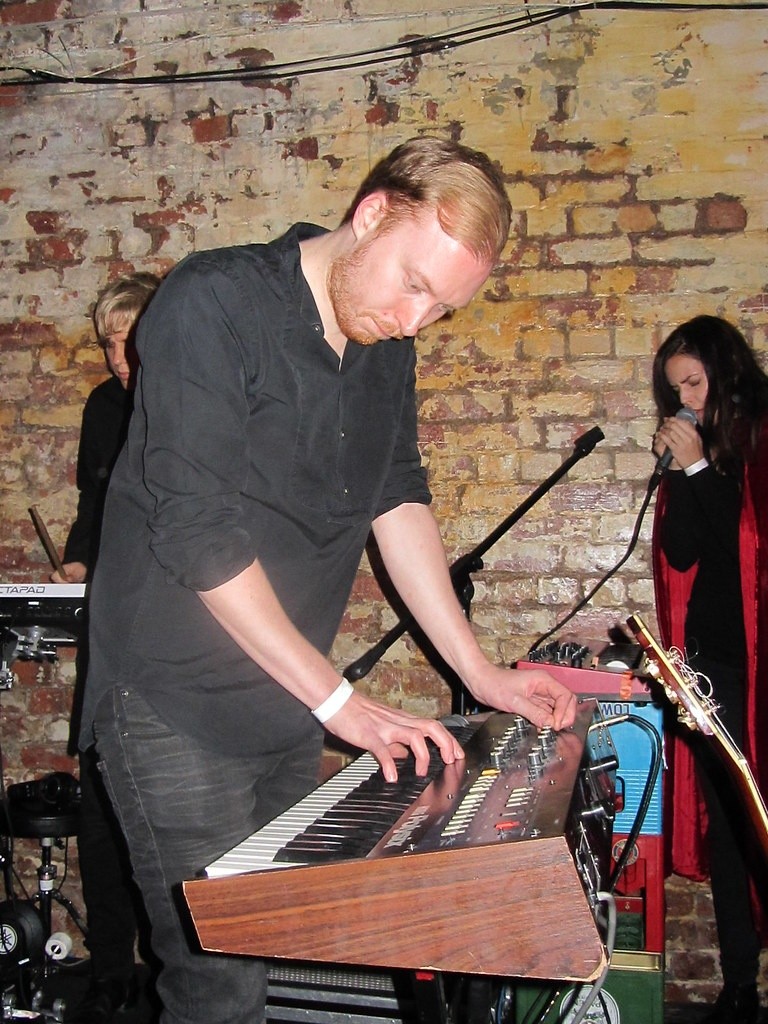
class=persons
[49,273,161,1024]
[79,136,578,1024]
[649,316,768,1023]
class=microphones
[647,407,698,492]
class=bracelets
[311,678,354,722]
[684,458,708,476]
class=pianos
[181,695,619,985]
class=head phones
[7,772,79,810]
